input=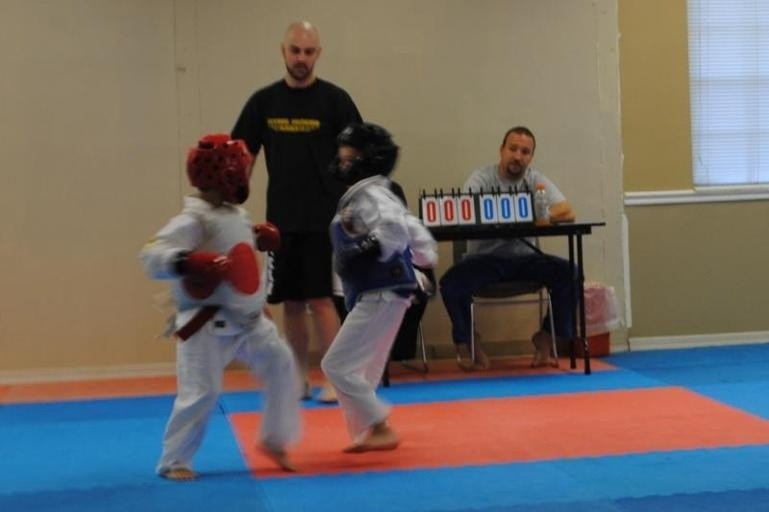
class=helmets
[327,121,398,184]
[187,134,255,204]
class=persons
[440,127,583,372]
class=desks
[379,219,606,392]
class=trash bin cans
[576,287,610,357]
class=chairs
[444,215,563,371]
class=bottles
[532,183,550,227]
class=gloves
[173,249,232,298]
[254,222,281,251]
[334,235,381,278]
